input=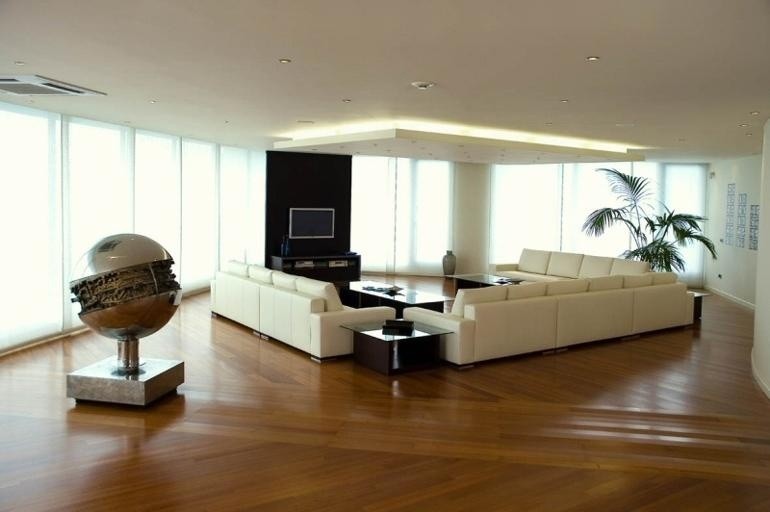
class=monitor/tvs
[289,207,335,239]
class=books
[382,319,414,330]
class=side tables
[339,320,454,376]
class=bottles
[281,232,287,256]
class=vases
[443,250,456,281]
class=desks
[271,255,361,286]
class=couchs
[489,248,650,282]
[403,272,694,370]
[210,260,396,364]
